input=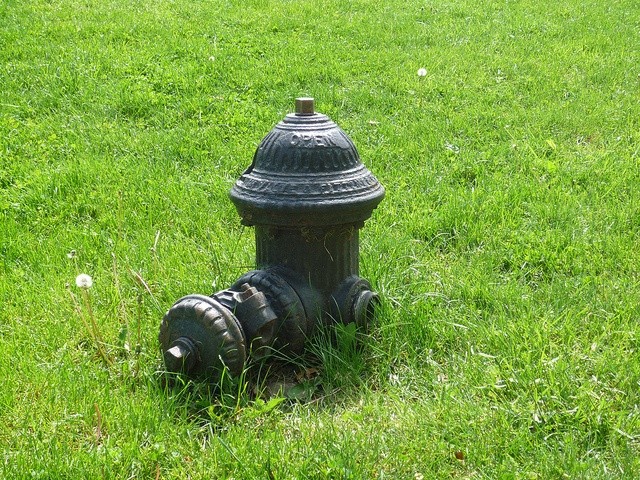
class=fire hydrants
[158,97,385,389]
